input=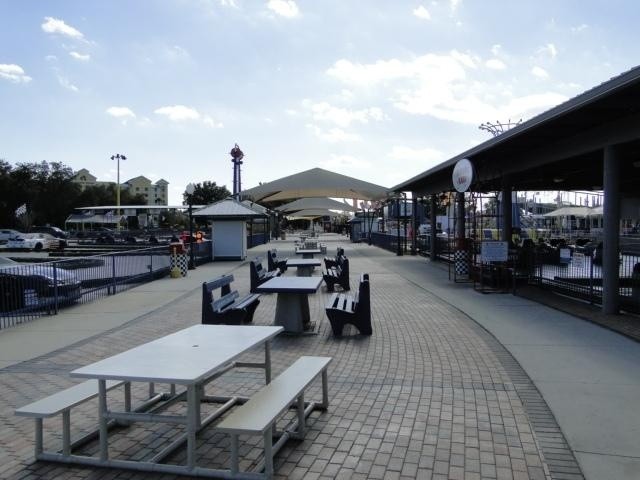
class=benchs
[214,356,332,475]
[14,378,155,460]
[197,247,374,341]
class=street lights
[112,153,126,233]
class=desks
[71,326,287,473]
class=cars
[79,228,195,245]
[1,224,70,251]
[0,257,81,311]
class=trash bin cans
[170,242,188,276]
[281,230,286,240]
[455,238,473,275]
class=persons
[148,235,158,243]
[272,253,287,274]
[171,235,179,242]
